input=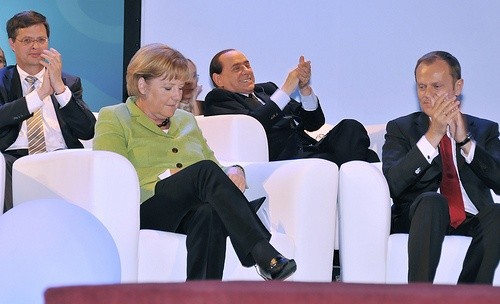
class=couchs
[338,161,500,286]
[0,112,338,304]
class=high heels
[259,252,297,281]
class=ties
[24,76,47,156]
[440,134,467,229]
[249,93,263,110]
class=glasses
[13,36,48,47]
[187,74,199,82]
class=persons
[382,51,500,286]
[0,48,7,71]
[175,59,206,116]
[92,44,296,281]
[204,49,380,170]
[0,10,97,213]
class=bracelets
[458,132,473,147]
